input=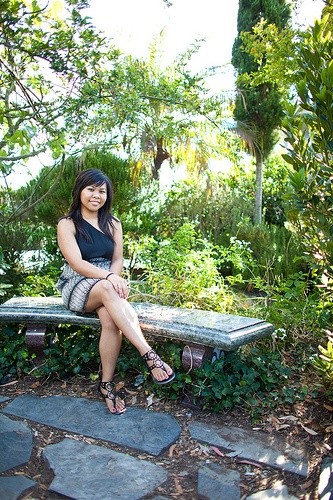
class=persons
[57,168,175,414]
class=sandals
[142,349,176,384]
[98,380,126,415]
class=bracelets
[106,273,114,280]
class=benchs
[0,297,274,410]
